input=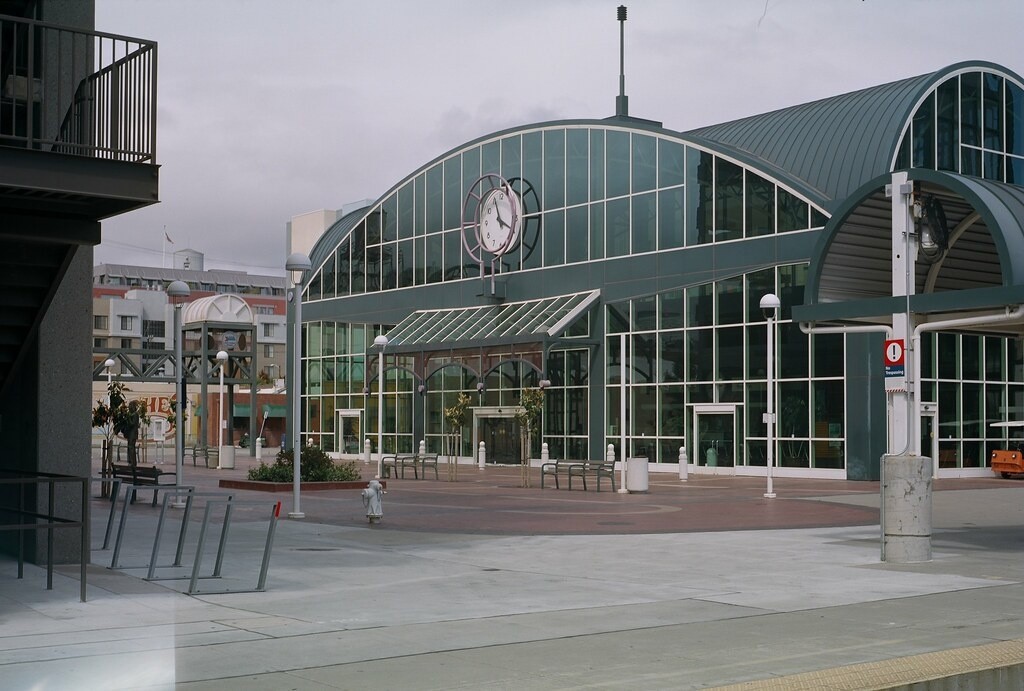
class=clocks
[480,186,522,253]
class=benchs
[109,462,176,507]
[541,458,615,492]
[184,446,219,468]
[381,453,439,480]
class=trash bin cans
[221,445,236,469]
[625,454,651,494]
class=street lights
[217,350,228,469]
[374,335,388,479]
[104,358,115,458]
[760,293,781,497]
